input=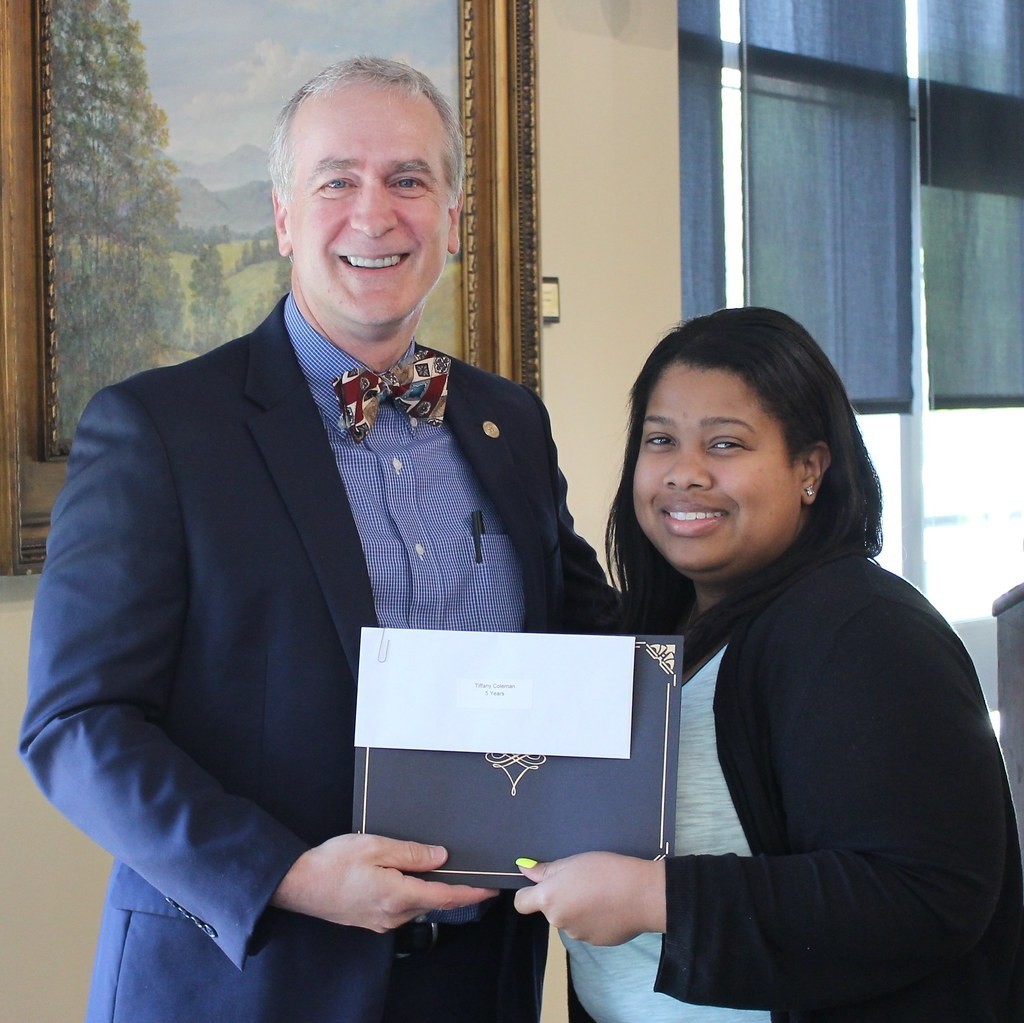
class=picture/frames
[0,0,544,581]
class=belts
[394,900,512,959]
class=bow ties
[333,349,454,443]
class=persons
[21,56,620,1023]
[514,304,1024,1023]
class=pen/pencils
[468,507,489,566]
